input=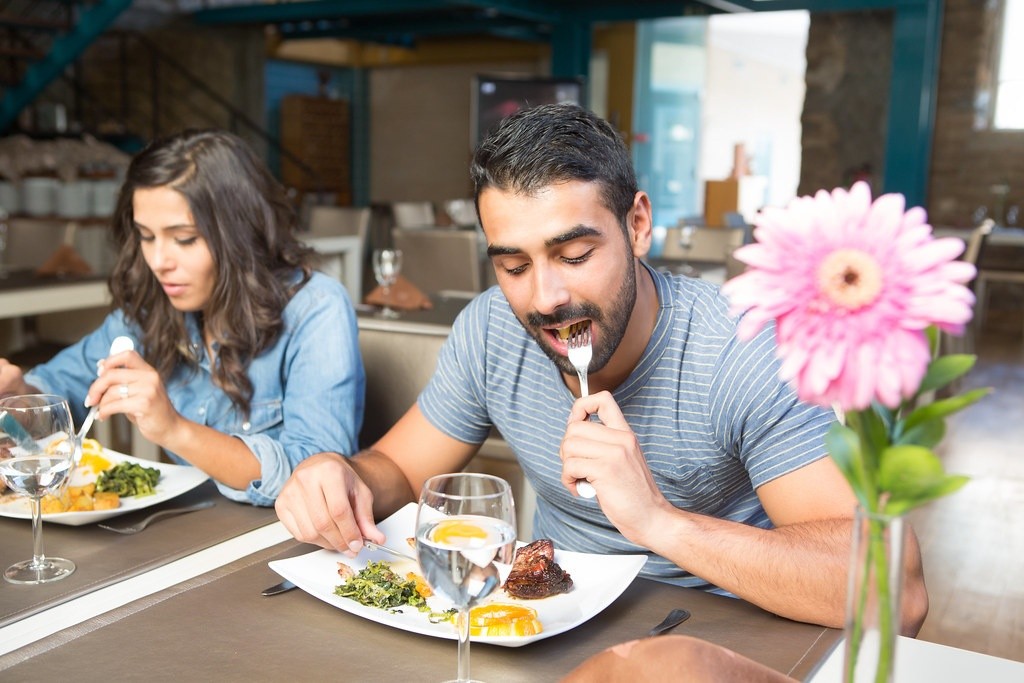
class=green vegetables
[96,459,160,497]
[333,559,457,624]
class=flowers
[719,182,997,683]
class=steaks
[504,539,573,599]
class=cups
[19,177,120,219]
[706,179,738,227]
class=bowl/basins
[443,199,478,230]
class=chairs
[0,194,679,336]
[339,316,453,451]
[954,216,1024,333]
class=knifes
[261,578,297,597]
[0,408,44,458]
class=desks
[919,225,1024,332]
[1,262,112,370]
[661,224,746,279]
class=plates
[1,430,211,526]
[557,636,801,683]
[268,501,649,648]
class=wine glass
[371,247,405,320]
[413,472,519,681]
[0,394,77,587]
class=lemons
[79,437,103,454]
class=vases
[839,500,909,682]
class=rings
[120,384,129,399]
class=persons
[272,102,931,639]
[0,127,369,509]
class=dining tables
[0,430,1024,683]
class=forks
[55,335,134,499]
[565,319,595,497]
[97,500,220,533]
